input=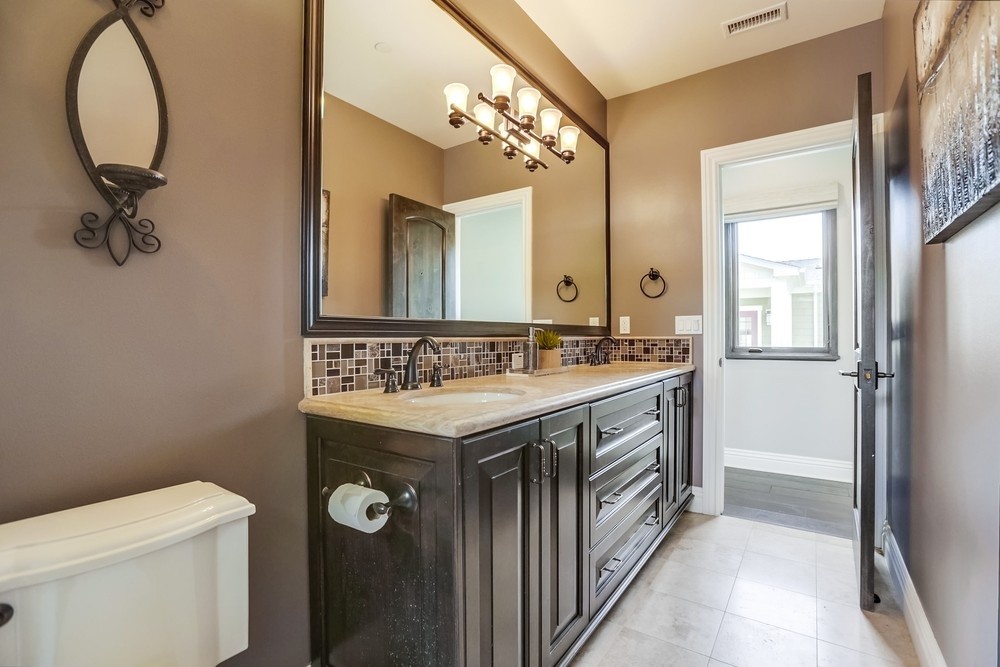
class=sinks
[606,363,685,371]
[403,387,527,405]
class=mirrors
[66,0,171,268]
[301,0,610,330]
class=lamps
[444,78,549,174]
[476,63,580,166]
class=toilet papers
[327,482,392,534]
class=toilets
[0,480,257,667]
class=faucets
[374,336,450,393]
[590,336,618,366]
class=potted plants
[528,333,562,369]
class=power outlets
[590,316,599,327]
[619,316,631,334]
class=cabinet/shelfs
[464,406,594,667]
[586,382,669,621]
[663,372,695,527]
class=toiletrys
[523,326,544,374]
